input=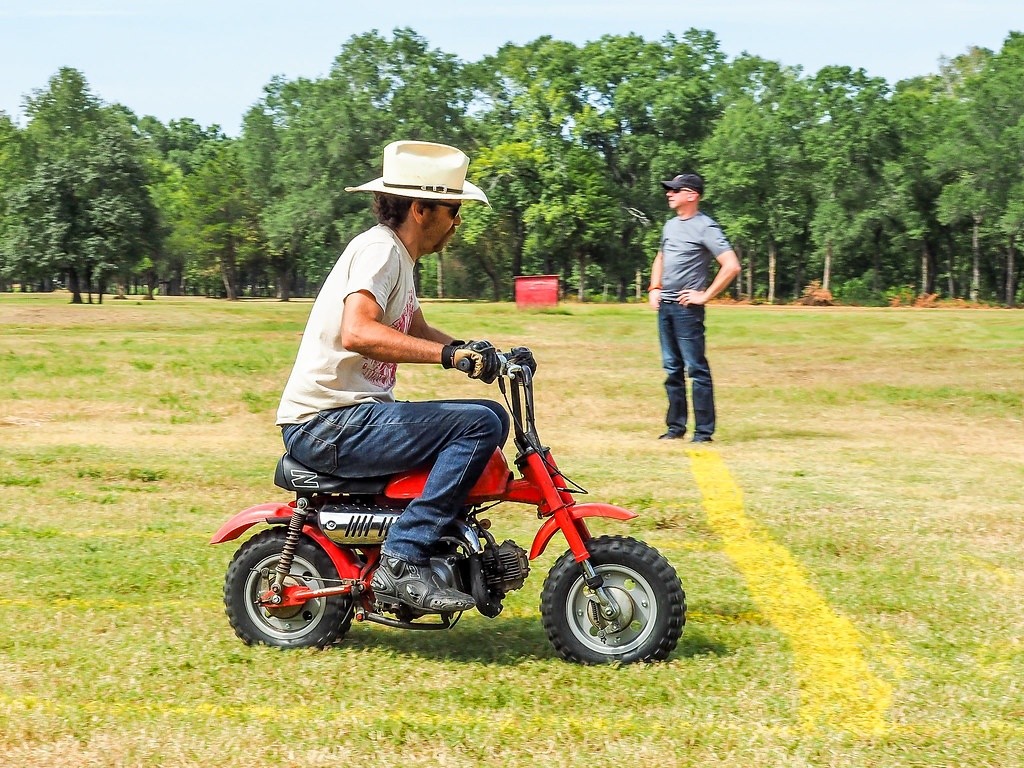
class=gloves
[495,343,537,382]
[441,340,501,385]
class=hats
[344,141,493,209]
[660,174,705,195]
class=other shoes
[691,439,711,444]
[659,434,684,441]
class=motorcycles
[205,356,690,668]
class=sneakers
[370,554,476,611]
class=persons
[648,173,742,443]
[272,141,537,613]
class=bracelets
[647,284,664,292]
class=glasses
[672,188,692,193]
[425,200,462,217]
